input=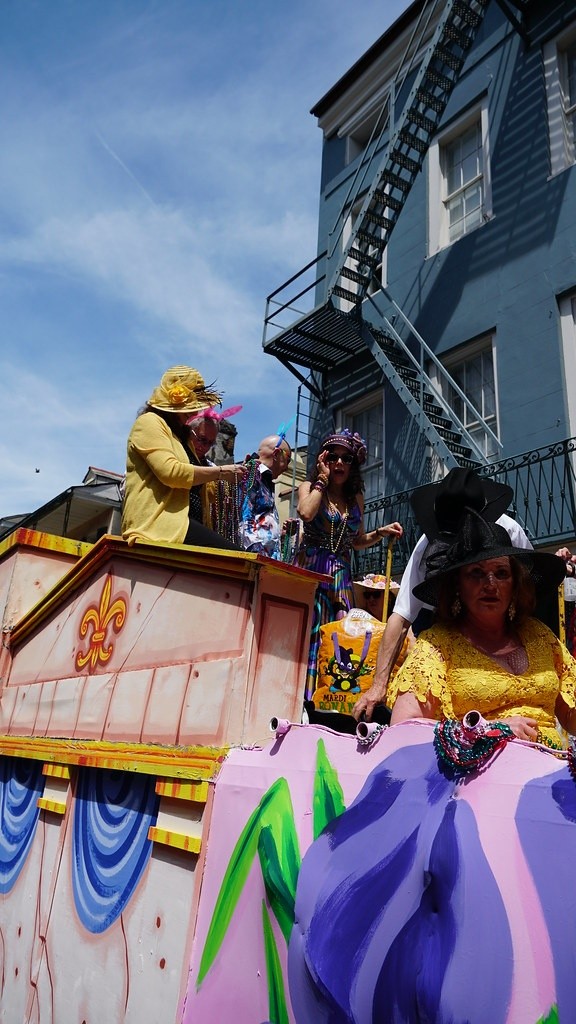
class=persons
[184,412,230,537]
[119,366,247,550]
[386,522,576,758]
[231,435,300,560]
[351,468,535,722]
[294,428,402,701]
[554,547,576,578]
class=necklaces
[208,459,259,548]
[283,518,304,564]
[326,489,348,553]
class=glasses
[192,430,216,447]
[326,453,354,465]
[363,590,384,599]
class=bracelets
[314,473,328,492]
[376,527,384,539]
[566,563,575,578]
[218,466,222,479]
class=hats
[145,366,224,413]
[320,428,367,462]
[409,467,566,609]
[353,574,400,607]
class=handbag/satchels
[310,618,410,716]
